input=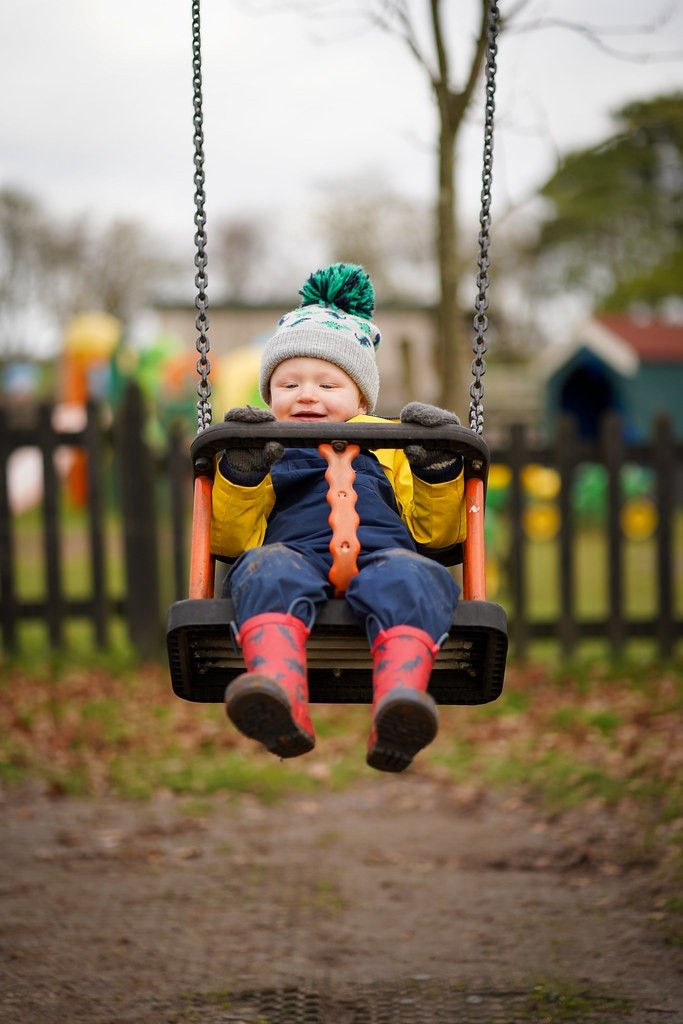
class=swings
[163,0,510,707]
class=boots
[225,597,316,760]
[365,613,450,773]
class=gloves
[223,404,285,483]
[400,401,461,470]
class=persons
[210,263,466,773]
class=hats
[259,262,383,415]
